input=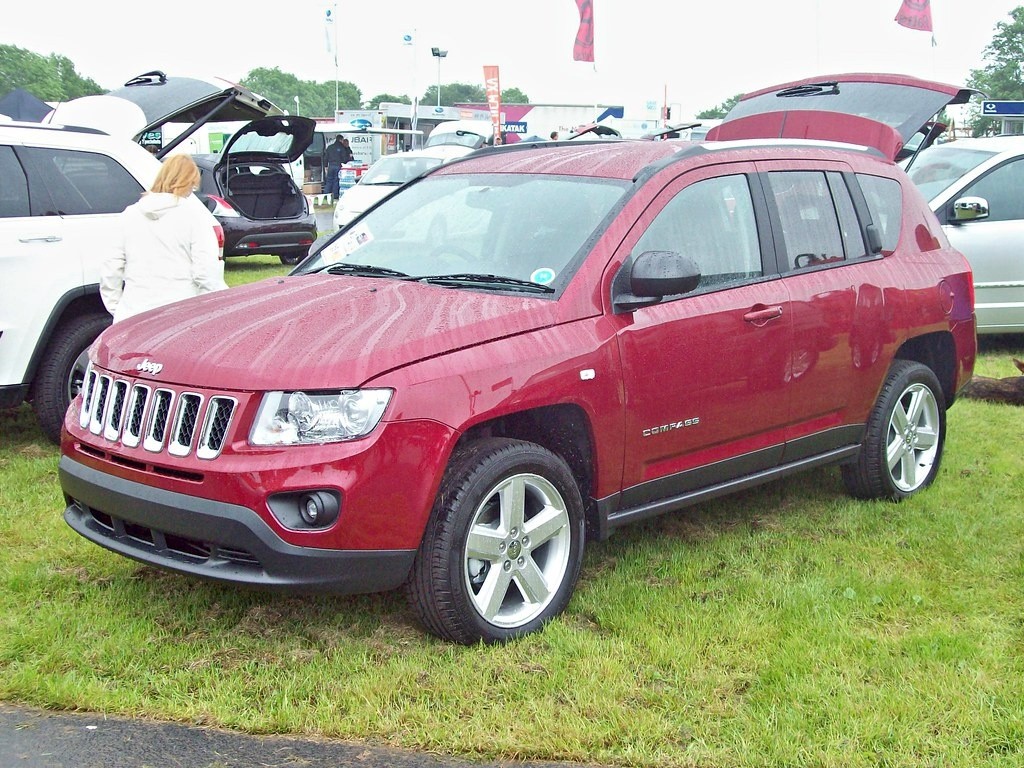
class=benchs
[303,192,332,207]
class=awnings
[303,122,424,169]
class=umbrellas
[513,135,549,142]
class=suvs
[1,71,274,447]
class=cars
[163,113,319,268]
[56,74,977,646]
[305,101,713,241]
[874,134,1023,334]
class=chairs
[259,170,273,174]
[222,169,238,184]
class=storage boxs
[302,184,322,195]
[303,170,312,182]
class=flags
[572,0,594,61]
[483,65,501,146]
[893,0,935,31]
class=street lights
[324,8,340,111]
[432,47,448,113]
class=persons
[550,131,559,141]
[494,137,502,146]
[576,124,585,132]
[98,153,232,324]
[323,134,354,200]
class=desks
[341,165,373,179]
